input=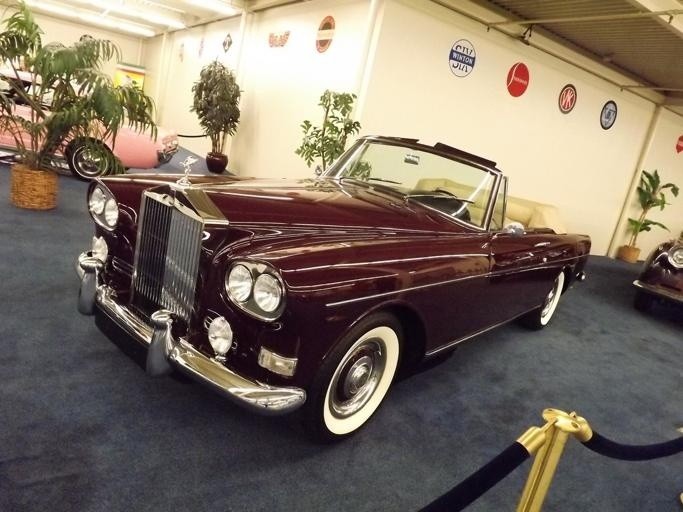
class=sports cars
[67,125,595,444]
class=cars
[0,63,182,185]
[630,229,682,321]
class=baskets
[9,160,59,210]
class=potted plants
[617,168,680,266]
[0,0,158,213]
[188,59,244,175]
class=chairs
[416,176,558,231]
